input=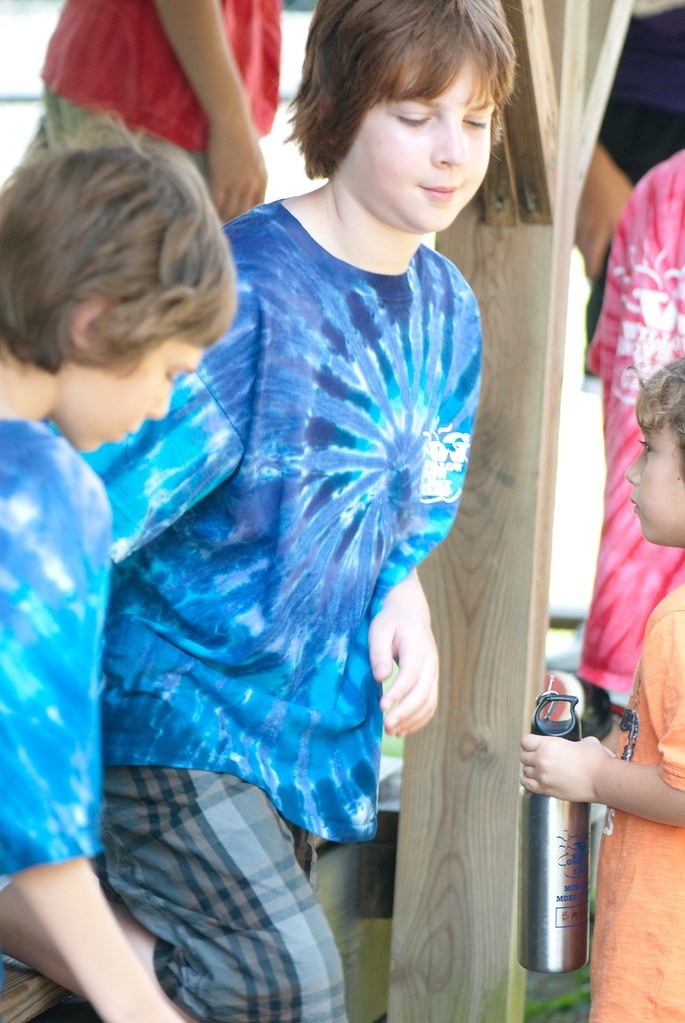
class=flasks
[516,675,590,974]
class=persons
[39,0,282,223]
[0,128,237,1023]
[45,0,516,1023]
[575,5,685,284]
[520,356,685,1023]
[577,147,685,696]
